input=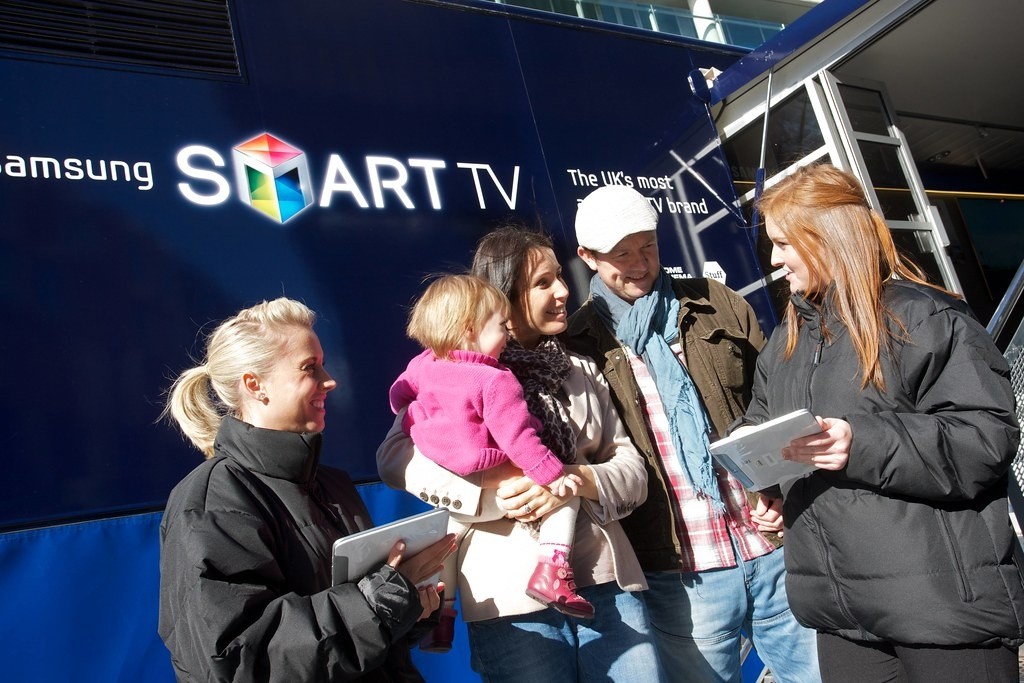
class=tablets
[709,408,827,493]
[331,508,449,590]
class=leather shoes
[419,608,457,653]
[525,555,595,620]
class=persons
[555,185,823,683]
[156,297,459,683]
[374,225,671,683]
[724,164,1024,683]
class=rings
[523,504,532,514]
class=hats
[574,185,658,254]
